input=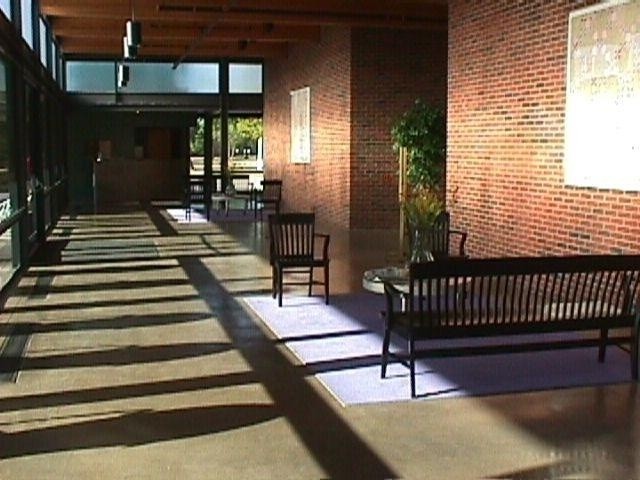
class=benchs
[379,252,640,399]
[186,172,256,215]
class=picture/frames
[289,84,313,166]
[562,0,640,193]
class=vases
[404,223,436,271]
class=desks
[361,268,474,313]
[211,192,251,217]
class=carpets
[165,206,279,225]
[241,282,640,411]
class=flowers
[397,178,450,263]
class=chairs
[266,211,332,307]
[407,208,470,262]
[182,181,210,222]
[252,179,283,221]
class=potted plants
[221,167,236,196]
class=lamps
[117,2,144,89]
